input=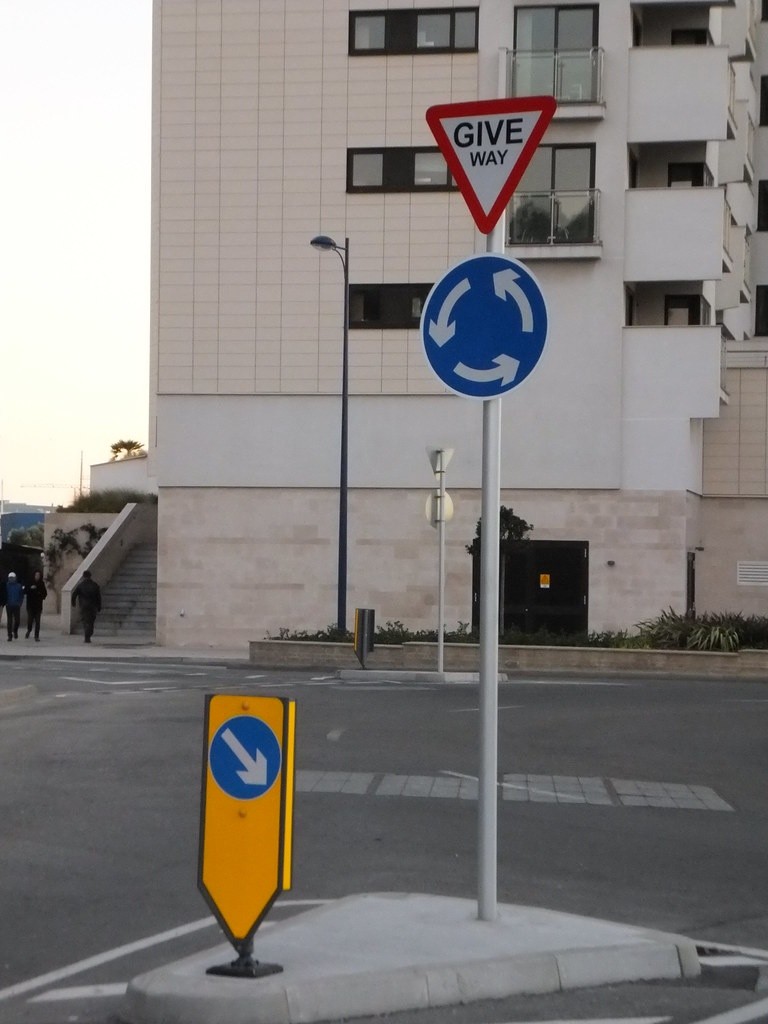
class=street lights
[311,236,349,644]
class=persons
[4,572,24,641]
[72,571,101,643]
[23,570,47,642]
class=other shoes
[26,630,31,638]
[85,637,90,642]
[35,635,40,641]
[14,632,18,638]
[7,635,12,641]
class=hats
[8,572,15,578]
